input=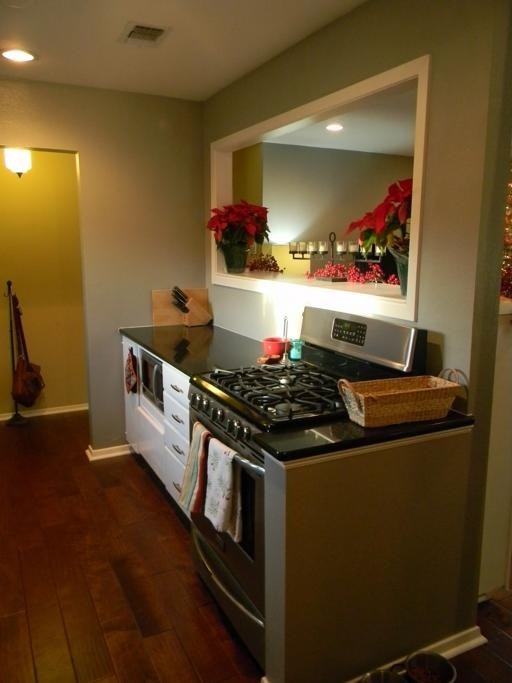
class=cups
[290,340,303,359]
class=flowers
[206,198,270,253]
[344,178,413,258]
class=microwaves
[139,351,163,413]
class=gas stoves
[186,359,349,439]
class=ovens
[184,393,266,668]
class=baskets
[337,367,462,428]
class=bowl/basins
[263,338,290,358]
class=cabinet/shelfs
[123,336,190,513]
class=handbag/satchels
[12,358,41,407]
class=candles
[289,240,386,254]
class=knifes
[170,287,189,313]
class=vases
[387,238,408,295]
[222,243,249,273]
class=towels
[178,421,243,544]
